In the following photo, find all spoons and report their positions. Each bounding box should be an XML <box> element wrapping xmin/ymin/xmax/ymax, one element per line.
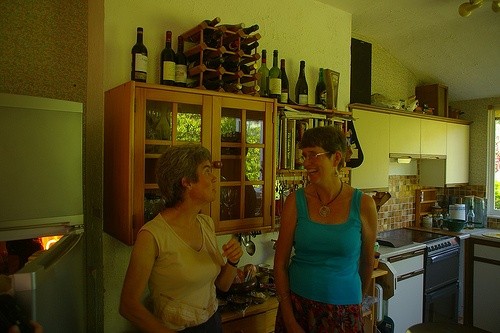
<box><xmin>245</xmin><ymin>235</ymin><xmax>256</xmax><ymax>256</ymax></box>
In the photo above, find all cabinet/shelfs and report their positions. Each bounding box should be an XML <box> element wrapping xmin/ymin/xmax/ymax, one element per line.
<box><xmin>220</xmin><ymin>269</ymin><xmax>388</xmax><ymax>333</ymax></box>
<box><xmin>181</xmin><ymin>23</ymin><xmax>262</xmax><ymax>96</ymax></box>
<box><xmin>461</xmin><ymin>237</ymin><xmax>500</xmax><ymax>333</ymax></box>
<box><xmin>104</xmin><ymin>80</ymin><xmax>278</xmax><ymax>247</ymax></box>
<box><xmin>273</xmin><ymin>103</ymin><xmax>352</xmax><ymax>227</ymax></box>
<box><xmin>348</xmin><ymin>103</ymin><xmax>474</xmax><ymax>190</ymax></box>
<box><xmin>381</xmin><ymin>249</ymin><xmax>425</xmax><ymax>333</ymax></box>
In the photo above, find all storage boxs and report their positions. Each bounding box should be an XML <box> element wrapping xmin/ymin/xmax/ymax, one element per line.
<box><xmin>416</xmin><ymin>84</ymin><xmax>449</xmax><ymax>116</ymax></box>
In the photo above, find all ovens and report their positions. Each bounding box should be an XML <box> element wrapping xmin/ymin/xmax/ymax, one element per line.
<box><xmin>422</xmin><ymin>248</ymin><xmax>459</xmax><ymax>333</ymax></box>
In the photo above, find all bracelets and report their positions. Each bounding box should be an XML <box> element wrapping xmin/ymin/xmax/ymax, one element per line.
<box><xmin>227</xmin><ymin>258</ymin><xmax>240</xmax><ymax>268</ymax></box>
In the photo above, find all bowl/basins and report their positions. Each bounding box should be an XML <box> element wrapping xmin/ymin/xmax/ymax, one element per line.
<box><xmin>446</xmin><ymin>219</ymin><xmax>466</xmax><ymax>231</ymax></box>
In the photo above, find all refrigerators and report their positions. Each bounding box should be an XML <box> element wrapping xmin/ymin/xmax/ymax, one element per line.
<box><xmin>0</xmin><ymin>92</ymin><xmax>87</xmax><ymax>333</ymax></box>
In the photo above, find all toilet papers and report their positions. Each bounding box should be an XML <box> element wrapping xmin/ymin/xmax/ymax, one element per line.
<box><xmin>448</xmin><ymin>203</ymin><xmax>466</xmax><ymax>220</ymax></box>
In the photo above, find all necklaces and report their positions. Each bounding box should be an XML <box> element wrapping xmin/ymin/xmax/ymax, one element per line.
<box><xmin>313</xmin><ymin>179</ymin><xmax>343</xmax><ymax>217</ymax></box>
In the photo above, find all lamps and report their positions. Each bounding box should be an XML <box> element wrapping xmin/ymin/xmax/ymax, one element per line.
<box><xmin>459</xmin><ymin>0</ymin><xmax>500</xmax><ymax>17</ymax></box>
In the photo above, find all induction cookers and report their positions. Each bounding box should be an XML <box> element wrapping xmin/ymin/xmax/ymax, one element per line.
<box><xmin>375</xmin><ymin>227</ymin><xmax>459</xmax><ymax>257</ymax></box>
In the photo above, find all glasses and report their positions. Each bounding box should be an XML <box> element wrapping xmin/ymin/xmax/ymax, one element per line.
<box><xmin>301</xmin><ymin>151</ymin><xmax>334</xmax><ymax>161</ymax></box>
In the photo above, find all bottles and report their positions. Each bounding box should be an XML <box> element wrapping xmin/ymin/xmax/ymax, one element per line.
<box><xmin>314</xmin><ymin>68</ymin><xmax>327</xmax><ymax>107</ymax></box>
<box><xmin>466</xmin><ymin>206</ymin><xmax>476</xmax><ymax>228</ymax></box>
<box><xmin>258</xmin><ymin>50</ymin><xmax>270</xmax><ymax>98</ymax></box>
<box><xmin>187</xmin><ymin>17</ymin><xmax>261</xmax><ymax>96</ymax></box>
<box><xmin>130</xmin><ymin>26</ymin><xmax>148</xmax><ymax>83</ymax></box>
<box><xmin>295</xmin><ymin>60</ymin><xmax>308</xmax><ymax>105</ymax></box>
<box><xmin>280</xmin><ymin>59</ymin><xmax>290</xmax><ymax>103</ymax></box>
<box><xmin>175</xmin><ymin>35</ymin><xmax>187</xmax><ymax>86</ymax></box>
<box><xmin>268</xmin><ymin>49</ymin><xmax>282</xmax><ymax>100</ymax></box>
<box><xmin>159</xmin><ymin>30</ymin><xmax>175</xmax><ymax>85</ymax></box>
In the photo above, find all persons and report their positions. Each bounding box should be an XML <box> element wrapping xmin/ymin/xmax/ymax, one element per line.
<box><xmin>273</xmin><ymin>126</ymin><xmax>378</xmax><ymax>333</ymax></box>
<box><xmin>118</xmin><ymin>144</ymin><xmax>243</xmax><ymax>333</ymax></box>
